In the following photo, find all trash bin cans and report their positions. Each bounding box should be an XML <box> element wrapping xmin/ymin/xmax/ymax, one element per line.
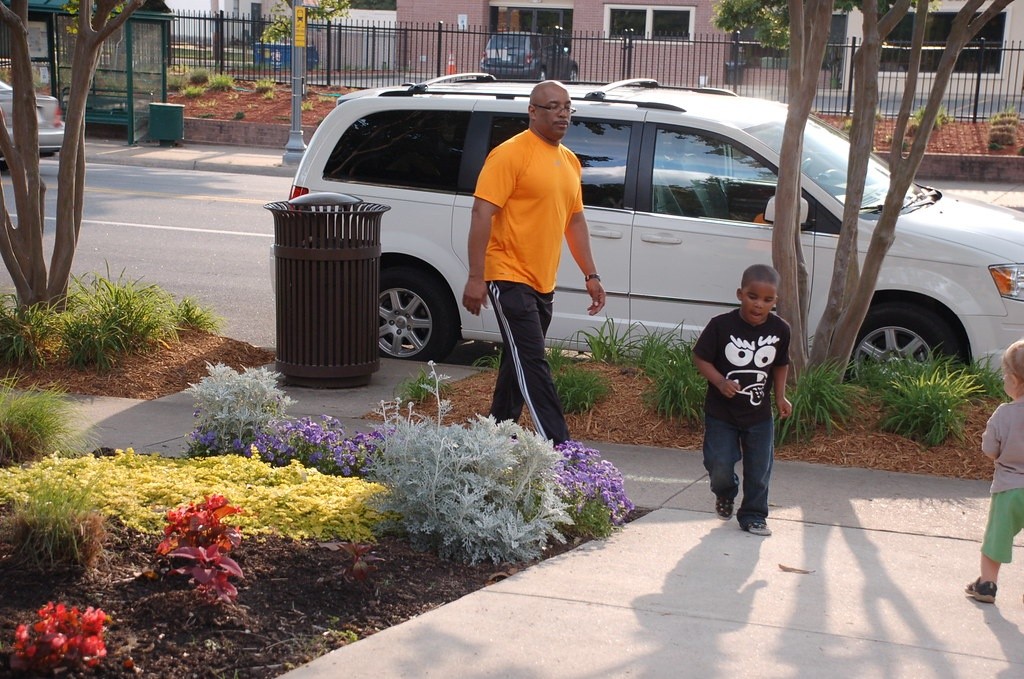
<box><xmin>263</xmin><ymin>190</ymin><xmax>393</xmax><ymax>392</ymax></box>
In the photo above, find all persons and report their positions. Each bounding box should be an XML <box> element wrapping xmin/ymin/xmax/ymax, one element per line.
<box><xmin>461</xmin><ymin>81</ymin><xmax>605</xmax><ymax>446</ymax></box>
<box><xmin>963</xmin><ymin>339</ymin><xmax>1024</xmax><ymax>604</ymax></box>
<box><xmin>691</xmin><ymin>265</ymin><xmax>793</xmax><ymax>535</ymax></box>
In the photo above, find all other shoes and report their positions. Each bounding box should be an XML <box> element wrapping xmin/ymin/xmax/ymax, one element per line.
<box><xmin>747</xmin><ymin>522</ymin><xmax>772</xmax><ymax>535</ymax></box>
<box><xmin>964</xmin><ymin>577</ymin><xmax>998</xmax><ymax>603</ymax></box>
<box><xmin>715</xmin><ymin>473</ymin><xmax>739</xmax><ymax>519</ymax></box>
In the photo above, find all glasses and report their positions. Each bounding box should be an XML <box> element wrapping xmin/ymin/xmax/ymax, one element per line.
<box><xmin>533</xmin><ymin>103</ymin><xmax>576</xmax><ymax>114</ymax></box>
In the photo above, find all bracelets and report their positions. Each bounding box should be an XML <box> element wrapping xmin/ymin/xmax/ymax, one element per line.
<box><xmin>585</xmin><ymin>273</ymin><xmax>602</xmax><ymax>282</ymax></box>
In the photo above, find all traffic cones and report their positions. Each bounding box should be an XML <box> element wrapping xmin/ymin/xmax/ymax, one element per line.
<box><xmin>445</xmin><ymin>52</ymin><xmax>456</xmax><ymax>75</ymax></box>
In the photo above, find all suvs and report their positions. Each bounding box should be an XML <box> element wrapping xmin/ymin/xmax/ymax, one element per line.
<box><xmin>287</xmin><ymin>72</ymin><xmax>1023</xmax><ymax>386</ymax></box>
<box><xmin>482</xmin><ymin>30</ymin><xmax>579</xmax><ymax>83</ymax></box>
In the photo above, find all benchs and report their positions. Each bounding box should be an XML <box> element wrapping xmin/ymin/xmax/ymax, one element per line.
<box><xmin>61</xmin><ymin>85</ymin><xmax>153</xmax><ymax>137</ymax></box>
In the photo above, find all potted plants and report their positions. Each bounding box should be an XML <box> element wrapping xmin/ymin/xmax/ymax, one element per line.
<box><xmin>827</xmin><ymin>48</ymin><xmax>842</xmax><ymax>88</ymax></box>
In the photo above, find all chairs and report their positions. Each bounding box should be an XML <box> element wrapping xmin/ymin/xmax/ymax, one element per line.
<box><xmin>654</xmin><ymin>157</ymin><xmax>730</xmax><ymax>219</ymax></box>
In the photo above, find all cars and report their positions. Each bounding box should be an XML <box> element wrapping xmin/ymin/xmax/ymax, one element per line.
<box><xmin>0</xmin><ymin>80</ymin><xmax>65</xmax><ymax>170</ymax></box>
